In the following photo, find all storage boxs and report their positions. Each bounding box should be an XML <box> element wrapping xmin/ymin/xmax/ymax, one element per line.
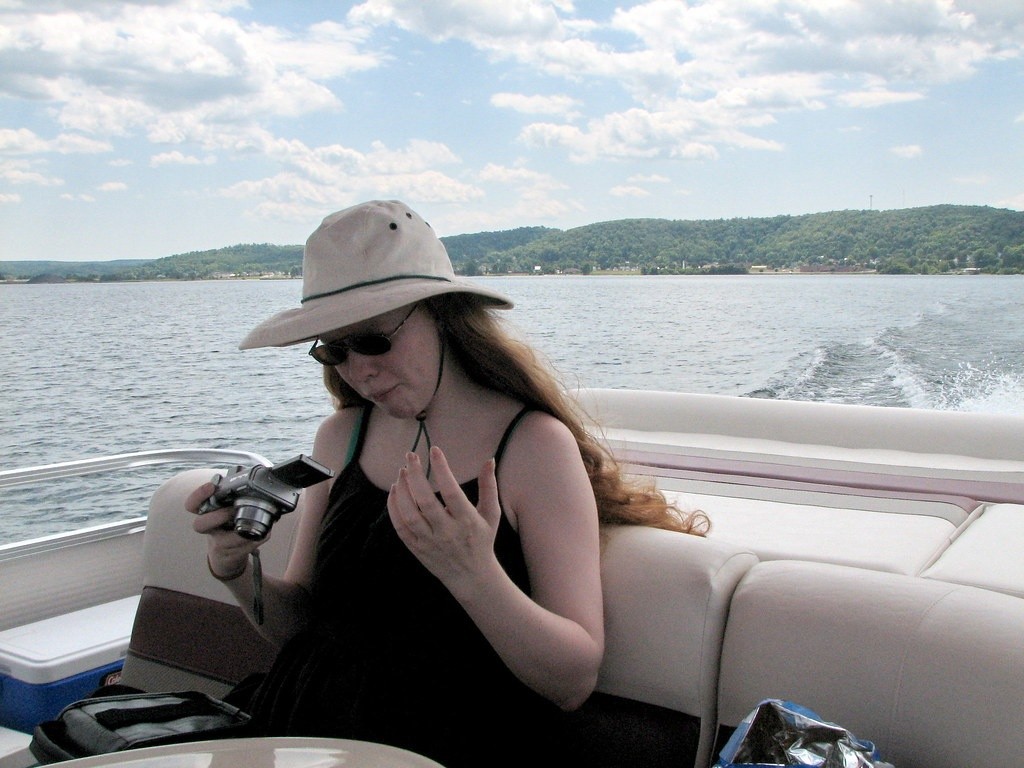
<box><xmin>0</xmin><ymin>593</ymin><xmax>140</xmax><ymax>734</ymax></box>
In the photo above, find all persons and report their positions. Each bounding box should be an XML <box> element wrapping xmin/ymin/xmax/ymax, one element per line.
<box><xmin>185</xmin><ymin>200</ymin><xmax>712</xmax><ymax>768</ymax></box>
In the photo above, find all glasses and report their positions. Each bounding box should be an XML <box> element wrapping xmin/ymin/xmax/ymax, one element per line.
<box><xmin>308</xmin><ymin>302</ymin><xmax>421</xmax><ymax>366</ymax></box>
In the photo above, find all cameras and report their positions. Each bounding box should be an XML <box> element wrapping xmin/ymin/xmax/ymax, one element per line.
<box><xmin>206</xmin><ymin>453</ymin><xmax>334</xmax><ymax>541</ymax></box>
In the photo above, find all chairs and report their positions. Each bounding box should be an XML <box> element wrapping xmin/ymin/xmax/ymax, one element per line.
<box><xmin>120</xmin><ymin>468</ymin><xmax>303</xmax><ymax>697</ymax></box>
<box><xmin>522</xmin><ymin>523</ymin><xmax>758</xmax><ymax>768</ymax></box>
<box><xmin>708</xmin><ymin>560</ymin><xmax>1024</xmax><ymax>768</ymax></box>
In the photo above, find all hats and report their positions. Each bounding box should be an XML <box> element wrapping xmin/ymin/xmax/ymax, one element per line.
<box><xmin>237</xmin><ymin>199</ymin><xmax>515</xmax><ymax>350</ymax></box>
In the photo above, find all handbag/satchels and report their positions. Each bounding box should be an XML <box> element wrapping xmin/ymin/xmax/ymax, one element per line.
<box><xmin>27</xmin><ymin>689</ymin><xmax>256</xmax><ymax>764</ymax></box>
<box><xmin>707</xmin><ymin>699</ymin><xmax>894</xmax><ymax>768</ymax></box>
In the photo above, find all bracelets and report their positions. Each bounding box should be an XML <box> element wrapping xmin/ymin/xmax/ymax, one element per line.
<box><xmin>207</xmin><ymin>554</ymin><xmax>245</xmax><ymax>580</ymax></box>
